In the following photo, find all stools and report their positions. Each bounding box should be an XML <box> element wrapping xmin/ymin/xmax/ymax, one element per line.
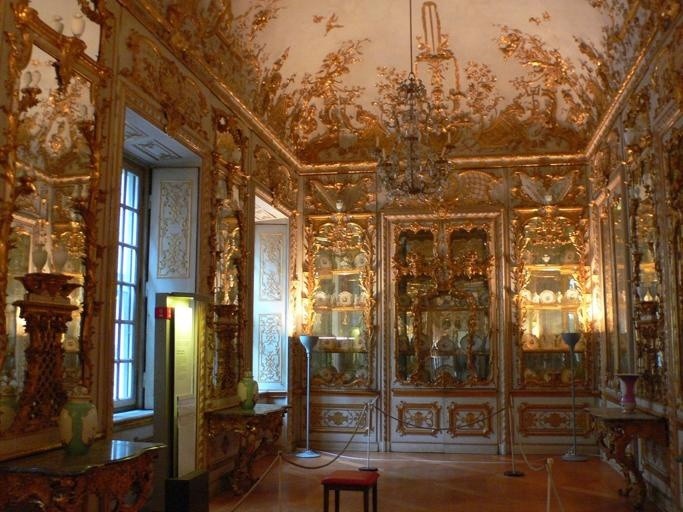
<box><xmin>318</xmin><ymin>468</ymin><xmax>379</xmax><ymax>512</ymax></box>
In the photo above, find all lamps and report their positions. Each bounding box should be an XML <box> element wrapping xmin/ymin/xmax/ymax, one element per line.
<box><xmin>559</xmin><ymin>330</ymin><xmax>589</xmax><ymax>460</ymax></box>
<box><xmin>372</xmin><ymin>1</ymin><xmax>459</xmax><ymax>208</ymax></box>
<box><xmin>292</xmin><ymin>334</ymin><xmax>321</xmax><ymax>459</ymax></box>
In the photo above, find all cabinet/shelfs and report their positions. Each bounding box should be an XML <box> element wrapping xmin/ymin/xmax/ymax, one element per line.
<box><xmin>306</xmin><ymin>268</ymin><xmax>368</xmax><ymax>354</ymax></box>
<box><xmin>518</xmin><ymin>262</ymin><xmax>591</xmax><ymax>354</ymax></box>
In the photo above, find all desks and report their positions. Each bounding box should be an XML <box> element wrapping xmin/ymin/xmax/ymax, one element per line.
<box><xmin>588</xmin><ymin>407</ymin><xmax>668</xmax><ymax>511</ymax></box>
<box><xmin>0</xmin><ymin>436</ymin><xmax>170</xmax><ymax>511</ymax></box>
<box><xmin>203</xmin><ymin>400</ymin><xmax>292</xmax><ymax>498</ymax></box>
